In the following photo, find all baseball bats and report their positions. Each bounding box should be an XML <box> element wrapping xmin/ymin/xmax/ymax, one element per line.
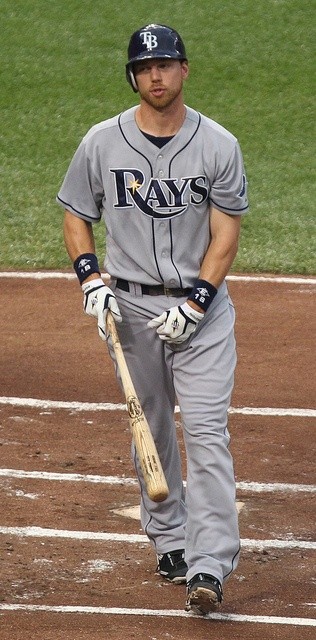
<box><xmin>106</xmin><ymin>311</ymin><xmax>170</xmax><ymax>503</ymax></box>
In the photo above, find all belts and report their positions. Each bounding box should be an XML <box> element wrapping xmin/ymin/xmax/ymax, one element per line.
<box><xmin>115</xmin><ymin>279</ymin><xmax>193</xmax><ymax>298</ymax></box>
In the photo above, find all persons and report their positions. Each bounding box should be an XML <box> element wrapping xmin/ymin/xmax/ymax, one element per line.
<box><xmin>55</xmin><ymin>23</ymin><xmax>251</xmax><ymax>615</ymax></box>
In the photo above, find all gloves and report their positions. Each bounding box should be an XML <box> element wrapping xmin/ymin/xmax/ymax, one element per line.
<box><xmin>83</xmin><ymin>279</ymin><xmax>122</xmax><ymax>342</ymax></box>
<box><xmin>147</xmin><ymin>301</ymin><xmax>204</xmax><ymax>345</ymax></box>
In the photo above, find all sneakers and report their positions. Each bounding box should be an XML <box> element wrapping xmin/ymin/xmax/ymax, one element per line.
<box><xmin>185</xmin><ymin>573</ymin><xmax>223</xmax><ymax>616</ymax></box>
<box><xmin>156</xmin><ymin>549</ymin><xmax>188</xmax><ymax>584</ymax></box>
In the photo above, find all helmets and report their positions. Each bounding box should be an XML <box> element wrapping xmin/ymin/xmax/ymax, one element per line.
<box><xmin>125</xmin><ymin>24</ymin><xmax>185</xmax><ymax>93</ymax></box>
